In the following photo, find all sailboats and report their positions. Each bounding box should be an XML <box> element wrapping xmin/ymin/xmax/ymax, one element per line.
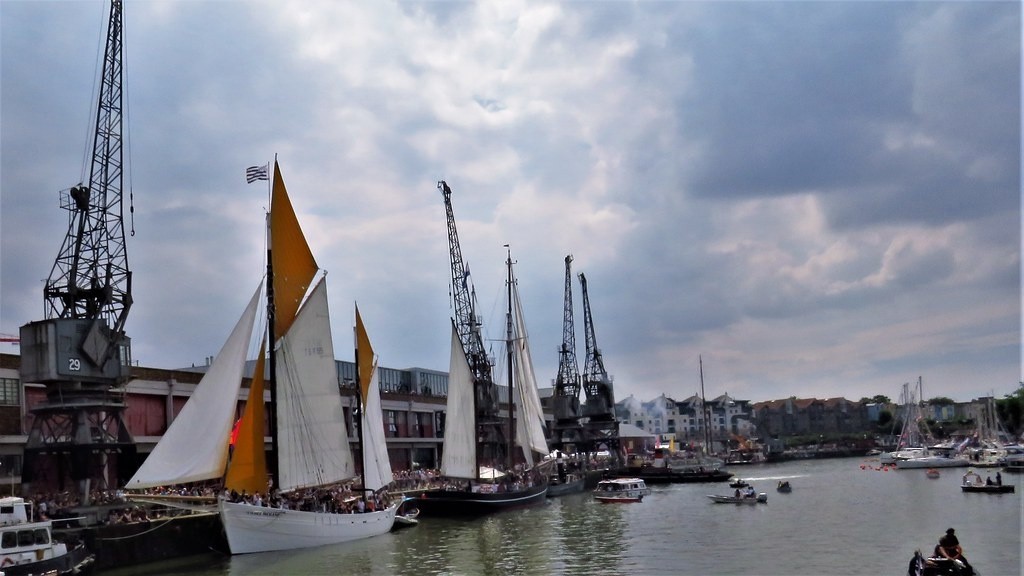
<box><xmin>116</xmin><ymin>152</ymin><xmax>735</xmax><ymax>554</ymax></box>
<box><xmin>880</xmin><ymin>376</ymin><xmax>1024</xmax><ymax>473</ymax></box>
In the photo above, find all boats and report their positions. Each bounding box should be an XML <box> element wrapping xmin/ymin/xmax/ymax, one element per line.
<box><xmin>706</xmin><ymin>492</ymin><xmax>767</xmax><ymax>504</ymax></box>
<box><xmin>729</xmin><ymin>483</ymin><xmax>749</xmax><ymax>488</ymax></box>
<box><xmin>592</xmin><ymin>478</ymin><xmax>651</xmax><ymax>502</ymax></box>
<box><xmin>0</xmin><ymin>494</ymin><xmax>221</xmax><ymax>575</ymax></box>
<box><xmin>865</xmin><ymin>449</ymin><xmax>881</xmax><ymax>456</ymax></box>
<box><xmin>777</xmin><ymin>487</ymin><xmax>792</xmax><ymax>493</ymax></box>
<box><xmin>960</xmin><ymin>470</ymin><xmax>1015</xmax><ymax>494</ymax></box>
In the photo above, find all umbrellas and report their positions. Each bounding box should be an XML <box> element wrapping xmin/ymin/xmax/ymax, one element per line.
<box><xmin>544</xmin><ymin>449</ymin><xmax>569</xmax><ymax>471</ymax></box>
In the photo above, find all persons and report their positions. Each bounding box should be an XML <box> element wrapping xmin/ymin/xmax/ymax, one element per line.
<box><xmin>986</xmin><ymin>477</ymin><xmax>993</xmax><ymax>485</ymax></box>
<box><xmin>933</xmin><ymin>528</ymin><xmax>962</xmax><ymax>561</ymax></box>
<box><xmin>1</xmin><ymin>485</ymin><xmax>217</xmax><ymax>526</ymax></box>
<box><xmin>352</xmin><ymin>469</ymin><xmax>441</xmax><ymax>491</ymax></box>
<box><xmin>444</xmin><ymin>461</ymin><xmax>531</xmax><ymax>495</ymax></box>
<box><xmin>743</xmin><ymin>486</ymin><xmax>756</xmax><ymax>499</ymax></box>
<box><xmin>224</xmin><ymin>486</ymin><xmax>395</xmax><ymax>514</ymax></box>
<box><xmin>995</xmin><ymin>472</ymin><xmax>1002</xmax><ymax>485</ymax></box>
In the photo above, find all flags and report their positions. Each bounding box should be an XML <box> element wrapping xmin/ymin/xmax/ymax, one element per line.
<box><xmin>246</xmin><ymin>165</ymin><xmax>269</xmax><ymax>184</ymax></box>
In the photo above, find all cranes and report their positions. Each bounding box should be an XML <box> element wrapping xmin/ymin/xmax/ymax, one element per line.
<box><xmin>19</xmin><ymin>0</ymin><xmax>139</xmax><ymax>504</ymax></box>
<box><xmin>550</xmin><ymin>248</ymin><xmax>623</xmax><ymax>461</ymax></box>
<box><xmin>436</xmin><ymin>178</ymin><xmax>503</xmax><ymax>426</ymax></box>
